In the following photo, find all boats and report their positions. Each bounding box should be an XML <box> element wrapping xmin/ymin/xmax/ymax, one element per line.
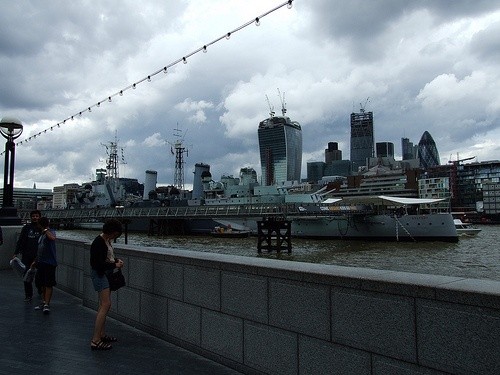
<box><xmin>209</xmin><ymin>229</ymin><xmax>251</xmax><ymax>238</ymax></box>
<box><xmin>456</xmin><ymin>228</ymin><xmax>482</xmax><ymax>236</ymax></box>
<box><xmin>453</xmin><ymin>218</ymin><xmax>472</xmax><ymax>229</ymax></box>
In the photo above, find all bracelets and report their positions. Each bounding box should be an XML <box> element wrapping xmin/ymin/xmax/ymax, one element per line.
<box><xmin>44</xmin><ymin>228</ymin><xmax>47</xmax><ymax>232</ymax></box>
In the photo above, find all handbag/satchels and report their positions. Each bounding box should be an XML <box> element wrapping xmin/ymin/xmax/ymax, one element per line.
<box><xmin>10</xmin><ymin>257</ymin><xmax>26</xmax><ymax>272</ymax></box>
<box><xmin>24</xmin><ymin>268</ymin><xmax>35</xmax><ymax>282</ymax></box>
<box><xmin>107</xmin><ymin>271</ymin><xmax>126</xmax><ymax>291</ymax></box>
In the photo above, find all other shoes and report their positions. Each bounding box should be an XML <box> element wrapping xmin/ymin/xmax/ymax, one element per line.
<box><xmin>35</xmin><ymin>302</ymin><xmax>46</xmax><ymax>310</ymax></box>
<box><xmin>23</xmin><ymin>295</ymin><xmax>33</xmax><ymax>303</ymax></box>
<box><xmin>43</xmin><ymin>304</ymin><xmax>50</xmax><ymax>312</ymax></box>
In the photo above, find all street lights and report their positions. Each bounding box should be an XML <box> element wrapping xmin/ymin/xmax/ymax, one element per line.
<box><xmin>0</xmin><ymin>115</ymin><xmax>24</xmax><ymax>227</ymax></box>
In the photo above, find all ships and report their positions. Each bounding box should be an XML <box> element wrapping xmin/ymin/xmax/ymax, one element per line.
<box><xmin>34</xmin><ymin>128</ymin><xmax>460</xmax><ymax>244</ymax></box>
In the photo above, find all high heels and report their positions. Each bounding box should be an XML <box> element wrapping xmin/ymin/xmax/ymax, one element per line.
<box><xmin>90</xmin><ymin>340</ymin><xmax>112</xmax><ymax>352</ymax></box>
<box><xmin>100</xmin><ymin>335</ymin><xmax>117</xmax><ymax>342</ymax></box>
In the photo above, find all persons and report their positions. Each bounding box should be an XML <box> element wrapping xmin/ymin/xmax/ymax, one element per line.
<box><xmin>12</xmin><ymin>211</ymin><xmax>43</xmax><ymax>302</ymax></box>
<box><xmin>31</xmin><ymin>217</ymin><xmax>56</xmax><ymax>313</ymax></box>
<box><xmin>90</xmin><ymin>218</ymin><xmax>123</xmax><ymax>351</ymax></box>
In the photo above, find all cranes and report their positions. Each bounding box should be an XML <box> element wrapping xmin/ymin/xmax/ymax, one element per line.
<box><xmin>359</xmin><ymin>96</ymin><xmax>370</xmax><ymax>113</ymax></box>
<box><xmin>263</xmin><ymin>88</ymin><xmax>288</xmax><ymax>118</ymax></box>
